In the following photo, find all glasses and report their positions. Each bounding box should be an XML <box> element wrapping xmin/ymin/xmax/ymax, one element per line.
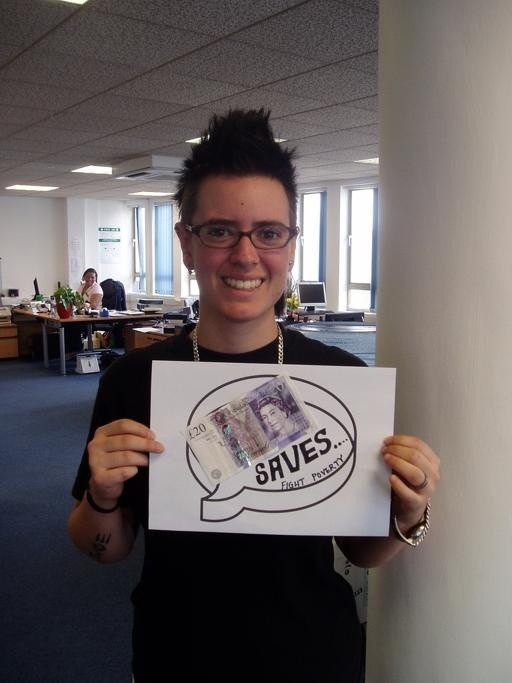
<box><xmin>185</xmin><ymin>221</ymin><xmax>297</xmax><ymax>250</ymax></box>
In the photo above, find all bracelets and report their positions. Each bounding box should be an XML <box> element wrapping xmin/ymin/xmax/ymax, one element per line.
<box><xmin>83</xmin><ymin>487</ymin><xmax>122</xmax><ymax>516</ymax></box>
<box><xmin>394</xmin><ymin>501</ymin><xmax>432</xmax><ymax>550</ymax></box>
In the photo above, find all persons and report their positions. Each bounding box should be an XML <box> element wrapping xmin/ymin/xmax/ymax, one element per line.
<box><xmin>67</xmin><ymin>266</ymin><xmax>105</xmax><ymax>361</ymax></box>
<box><xmin>255</xmin><ymin>396</ymin><xmax>298</xmax><ymax>437</ymax></box>
<box><xmin>66</xmin><ymin>101</ymin><xmax>442</xmax><ymax>681</ymax></box>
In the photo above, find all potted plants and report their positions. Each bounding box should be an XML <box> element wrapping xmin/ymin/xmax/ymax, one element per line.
<box><xmin>53</xmin><ymin>285</ymin><xmax>85</xmax><ymax>319</ymax></box>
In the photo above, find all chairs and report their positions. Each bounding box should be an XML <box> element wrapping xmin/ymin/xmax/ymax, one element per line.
<box><xmin>88</xmin><ymin>278</ymin><xmax>127</xmax><ymax>350</ymax></box>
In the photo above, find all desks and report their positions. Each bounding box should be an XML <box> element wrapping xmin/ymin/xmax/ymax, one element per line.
<box><xmin>12</xmin><ymin>306</ymin><xmax>178</xmax><ymax>376</ymax></box>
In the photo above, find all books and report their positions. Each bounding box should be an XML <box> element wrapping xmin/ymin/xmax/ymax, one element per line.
<box><xmin>132</xmin><ymin>326</ymin><xmax>160</xmax><ymax>334</ymax></box>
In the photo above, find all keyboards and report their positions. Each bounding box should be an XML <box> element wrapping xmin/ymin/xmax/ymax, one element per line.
<box><xmin>298</xmin><ymin>310</ymin><xmax>334</xmax><ymax>315</ymax></box>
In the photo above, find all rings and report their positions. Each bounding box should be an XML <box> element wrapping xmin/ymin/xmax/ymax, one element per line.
<box><xmin>414</xmin><ymin>472</ymin><xmax>430</xmax><ymax>489</ymax></box>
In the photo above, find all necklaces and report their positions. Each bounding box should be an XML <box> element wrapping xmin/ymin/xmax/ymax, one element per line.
<box><xmin>190</xmin><ymin>319</ymin><xmax>288</xmax><ymax>368</ymax></box>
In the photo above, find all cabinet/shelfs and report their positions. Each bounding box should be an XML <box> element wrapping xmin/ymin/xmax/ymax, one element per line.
<box><xmin>0</xmin><ymin>323</ymin><xmax>20</xmax><ymax>361</ymax></box>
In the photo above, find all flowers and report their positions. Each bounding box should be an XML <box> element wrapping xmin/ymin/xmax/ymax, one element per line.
<box><xmin>285</xmin><ymin>293</ymin><xmax>301</xmax><ymax>319</ymax></box>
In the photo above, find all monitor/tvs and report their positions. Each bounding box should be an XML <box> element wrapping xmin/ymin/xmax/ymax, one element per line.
<box><xmin>325</xmin><ymin>312</ymin><xmax>364</xmax><ymax>322</ymax></box>
<box><xmin>297</xmin><ymin>281</ymin><xmax>327</xmax><ymax>312</ymax></box>
<box><xmin>32</xmin><ymin>278</ymin><xmax>40</xmax><ymax>300</ymax></box>
